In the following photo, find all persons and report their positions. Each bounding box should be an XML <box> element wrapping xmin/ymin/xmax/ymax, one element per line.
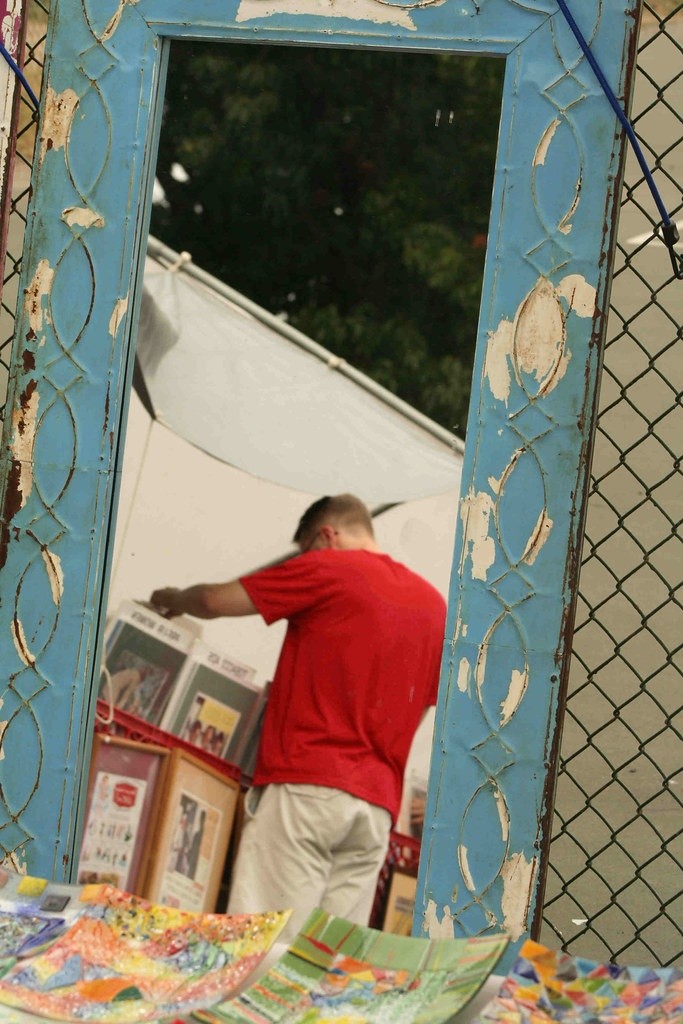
<box><xmin>152</xmin><ymin>493</ymin><xmax>449</xmax><ymax>930</ymax></box>
<box><xmin>101</xmin><ymin>666</ymin><xmax>146</xmax><ymax>709</ymax></box>
<box><xmin>171</xmin><ymin>810</ymin><xmax>207</xmax><ymax>879</ymax></box>
<box><xmin>186</xmin><ymin>722</ymin><xmax>226</xmax><ymax>754</ymax></box>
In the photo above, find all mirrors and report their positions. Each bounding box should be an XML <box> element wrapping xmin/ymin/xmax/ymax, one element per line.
<box><xmin>0</xmin><ymin>0</ymin><xmax>639</xmax><ymax>985</ymax></box>
